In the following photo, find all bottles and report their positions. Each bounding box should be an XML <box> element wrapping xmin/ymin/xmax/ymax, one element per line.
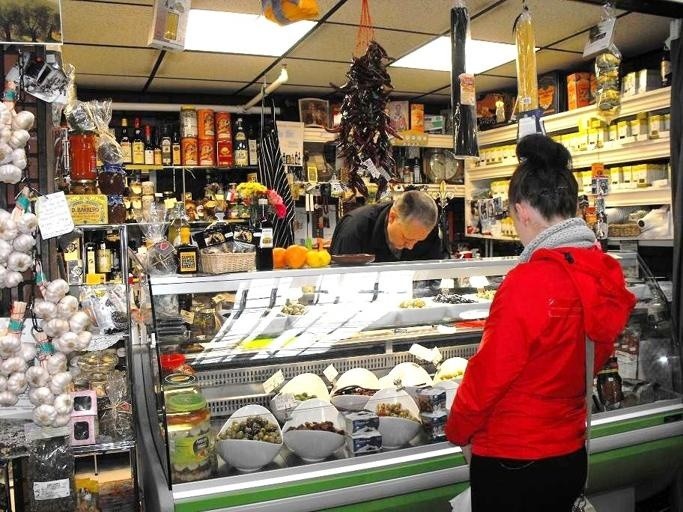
<box><xmin>226</xmin><ymin>183</ymin><xmax>239</xmax><ymax>202</ymax></box>
<box><xmin>160</xmin><ymin>123</ymin><xmax>172</xmax><ymax>164</ymax></box>
<box><xmin>613</xmin><ymin>328</ymin><xmax>639</xmax><ymax>377</ymax></box>
<box><xmin>120</xmin><ymin>118</ymin><xmax>132</xmax><ymax>164</ymax></box>
<box><xmin>132</xmin><ymin>118</ymin><xmax>145</xmax><ymax>164</ymax></box>
<box><xmin>215</xmin><ymin>111</ymin><xmax>231</xmax><ymax>140</ymax></box>
<box><xmin>182</xmin><ymin>136</ymin><xmax>196</xmax><ymax>166</ymax></box>
<box><xmin>90</xmin><ymin>229</ymin><xmax>97</xmax><ymax>244</ymax></box>
<box><xmin>122</xmin><ymin>197</ymin><xmax>129</xmax><ymax>222</ymax></box>
<box><xmin>108</xmin><ymin>195</ymin><xmax>126</xmax><ymax>223</ymax></box>
<box><xmin>254</xmin><ymin>199</ymin><xmax>273</xmax><ymax>270</ymax></box>
<box><xmin>180</xmin><ymin>105</ymin><xmax>197</xmax><ymax>137</ymax></box>
<box><xmin>175</xmin><ymin>227</ymin><xmax>198</xmax><ymax>272</ymax></box>
<box><xmin>413</xmin><ymin>157</ymin><xmax>420</xmax><ymax>183</ymax></box>
<box><xmin>144</xmin><ymin>124</ymin><xmax>154</xmax><ymax>164</ymax></box>
<box><xmin>312</xmin><ymin>193</ymin><xmax>325</xmax><ymax>238</ymax></box>
<box><xmin>142</xmin><ymin>182</ymin><xmax>154</xmax><ymax>195</ymax></box>
<box><xmin>197</xmin><ymin>139</ymin><xmax>215</xmax><ymax>167</ymax></box>
<box><xmin>239</xmin><ymin>202</ymin><xmax>250</xmax><ymax>218</ymax></box>
<box><xmin>129</xmin><ymin>196</ymin><xmax>142</xmax><ymax>223</ymax></box>
<box><xmin>234</xmin><ymin>118</ymin><xmax>249</xmax><ymax>166</ymax></box>
<box><xmin>98</xmin><ymin>164</ymin><xmax>126</xmax><ymax>195</ymax></box>
<box><xmin>96</xmin><ymin>241</ymin><xmax>110</xmax><ymax>281</ymax></box>
<box><xmin>164</xmin><ymin>192</ymin><xmax>173</xmax><ymax>200</ymax></box>
<box><xmin>248</xmin><ymin>127</ymin><xmax>258</xmax><ymax>165</ymax></box>
<box><xmin>87</xmin><ymin>245</ymin><xmax>95</xmax><ymax>274</ymax></box>
<box><xmin>403</xmin><ymin>165</ymin><xmax>411</xmax><ymax>182</ymax></box>
<box><xmin>227</xmin><ymin>203</ymin><xmax>238</xmax><ymax>219</ymax></box>
<box><xmin>661</xmin><ymin>40</ymin><xmax>672</xmax><ymax>85</ymax></box>
<box><xmin>152</xmin><ymin>127</ymin><xmax>162</xmax><ymax>164</ymax></box>
<box><xmin>172</xmin><ymin>125</ymin><xmax>181</xmax><ymax>165</ymax></box>
<box><xmin>130</xmin><ymin>182</ymin><xmax>141</xmax><ymax>196</ymax></box>
<box><xmin>198</xmin><ymin>106</ymin><xmax>213</xmax><ymax>139</ymax></box>
<box><xmin>217</xmin><ymin>140</ymin><xmax>232</xmax><ymax>166</ymax></box>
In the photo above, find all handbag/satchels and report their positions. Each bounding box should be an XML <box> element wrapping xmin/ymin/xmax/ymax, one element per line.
<box><xmin>573</xmin><ymin>334</ymin><xmax>596</xmax><ymax>512</ymax></box>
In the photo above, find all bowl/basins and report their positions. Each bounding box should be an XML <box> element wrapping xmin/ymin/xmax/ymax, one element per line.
<box><xmin>363</xmin><ymin>386</ymin><xmax>423</xmax><ymax>449</ymax></box>
<box><xmin>388</xmin><ymin>362</ymin><xmax>433</xmax><ymax>388</ymax></box>
<box><xmin>433</xmin><ymin>357</ymin><xmax>468</xmax><ymax>385</ymax></box>
<box><xmin>282</xmin><ymin>397</ymin><xmax>348</xmax><ymax>462</ymax></box>
<box><xmin>329</xmin><ymin>368</ymin><xmax>380</xmax><ymax>411</ymax></box>
<box><xmin>214</xmin><ymin>404</ymin><xmax>284</xmax><ymax>472</ymax></box>
<box><xmin>270</xmin><ymin>373</ymin><xmax>330</xmax><ymax>423</ymax></box>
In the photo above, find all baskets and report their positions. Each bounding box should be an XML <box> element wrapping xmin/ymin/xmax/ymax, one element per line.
<box><xmin>198</xmin><ymin>250</ymin><xmax>257</xmax><ymax>274</ymax></box>
<box><xmin>608</xmin><ymin>223</ymin><xmax>640</xmax><ymax>237</ymax></box>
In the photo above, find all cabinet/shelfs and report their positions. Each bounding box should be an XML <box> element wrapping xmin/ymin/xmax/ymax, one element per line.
<box><xmin>461</xmin><ymin>84</ymin><xmax>675</xmax><ymax>249</ymax></box>
<box><xmin>274</xmin><ymin>116</ymin><xmax>486</xmax><ymax>263</ymax></box>
<box><xmin>125</xmin><ymin>255</ymin><xmax>681</xmax><ymax>512</ymax></box>
<box><xmin>51</xmin><ymin>100</ymin><xmax>280</xmax><ymax>271</ymax></box>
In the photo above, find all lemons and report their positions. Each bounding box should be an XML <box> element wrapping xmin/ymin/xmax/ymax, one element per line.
<box><xmin>306</xmin><ymin>249</ymin><xmax>330</xmax><ymax>267</ymax></box>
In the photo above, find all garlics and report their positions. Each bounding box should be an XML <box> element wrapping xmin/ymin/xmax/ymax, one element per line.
<box><xmin>36</xmin><ymin>278</ymin><xmax>92</xmax><ymax>356</ymax></box>
<box><xmin>24</xmin><ymin>351</ymin><xmax>75</xmax><ymax>428</ymax></box>
<box><xmin>0</xmin><ymin>208</ymin><xmax>37</xmax><ymax>289</ymax></box>
<box><xmin>0</xmin><ymin>334</ymin><xmax>37</xmax><ymax>406</ymax></box>
<box><xmin>0</xmin><ymin>100</ymin><xmax>35</xmax><ymax>185</ymax></box>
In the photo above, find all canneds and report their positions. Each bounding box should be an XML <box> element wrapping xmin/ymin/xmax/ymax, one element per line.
<box><xmin>162</xmin><ymin>373</ymin><xmax>200</xmax><ymax>403</ymax></box>
<box><xmin>163</xmin><ymin>395</ymin><xmax>213</xmax><ymax>481</ymax></box>
<box><xmin>605</xmin><ymin>357</ymin><xmax>618</xmax><ymax>371</ymax></box>
<box><xmin>596</xmin><ymin>369</ymin><xmax>623</xmax><ymax>404</ymax></box>
<box><xmin>201</xmin><ymin>310</ymin><xmax>215</xmax><ymax>333</ymax></box>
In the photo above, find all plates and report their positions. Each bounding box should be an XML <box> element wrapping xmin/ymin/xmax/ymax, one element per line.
<box><xmin>331</xmin><ymin>253</ymin><xmax>375</xmax><ymax>267</ymax></box>
<box><xmin>459</xmin><ymin>308</ymin><xmax>490</xmax><ymax>320</ymax></box>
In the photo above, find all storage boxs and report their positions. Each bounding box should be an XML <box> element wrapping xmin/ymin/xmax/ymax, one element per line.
<box><xmin>605</xmin><ymin>222</ymin><xmax>643</xmax><ymax>238</ymax></box>
<box><xmin>567</xmin><ymin>73</ymin><xmax>590</xmax><ymax>111</ymax></box>
<box><xmin>536</xmin><ymin>68</ymin><xmax>564</xmax><ymax>118</ymax></box>
<box><xmin>196</xmin><ymin>245</ymin><xmax>256</xmax><ymax>276</ymax></box>
<box><xmin>423</xmin><ymin>115</ymin><xmax>444</xmax><ymax>135</ymax></box>
<box><xmin>385</xmin><ymin>101</ymin><xmax>408</xmax><ymax>133</ymax></box>
<box><xmin>410</xmin><ymin>103</ymin><xmax>423</xmax><ymax>134</ymax></box>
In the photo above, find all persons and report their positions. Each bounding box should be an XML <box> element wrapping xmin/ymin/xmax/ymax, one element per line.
<box><xmin>330</xmin><ymin>190</ymin><xmax>444</xmax><ymax>262</ymax></box>
<box><xmin>444</xmin><ymin>133</ymin><xmax>635</xmax><ymax>512</ymax></box>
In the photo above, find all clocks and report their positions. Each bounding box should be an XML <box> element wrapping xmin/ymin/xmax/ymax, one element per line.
<box><xmin>423</xmin><ymin>148</ymin><xmax>465</xmax><ymax>184</ymax></box>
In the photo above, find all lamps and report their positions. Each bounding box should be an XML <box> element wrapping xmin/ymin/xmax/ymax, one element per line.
<box><xmin>182</xmin><ymin>7</ymin><xmax>317</xmax><ymax>58</ymax></box>
<box><xmin>385</xmin><ymin>35</ymin><xmax>541</xmax><ymax>77</ymax></box>
